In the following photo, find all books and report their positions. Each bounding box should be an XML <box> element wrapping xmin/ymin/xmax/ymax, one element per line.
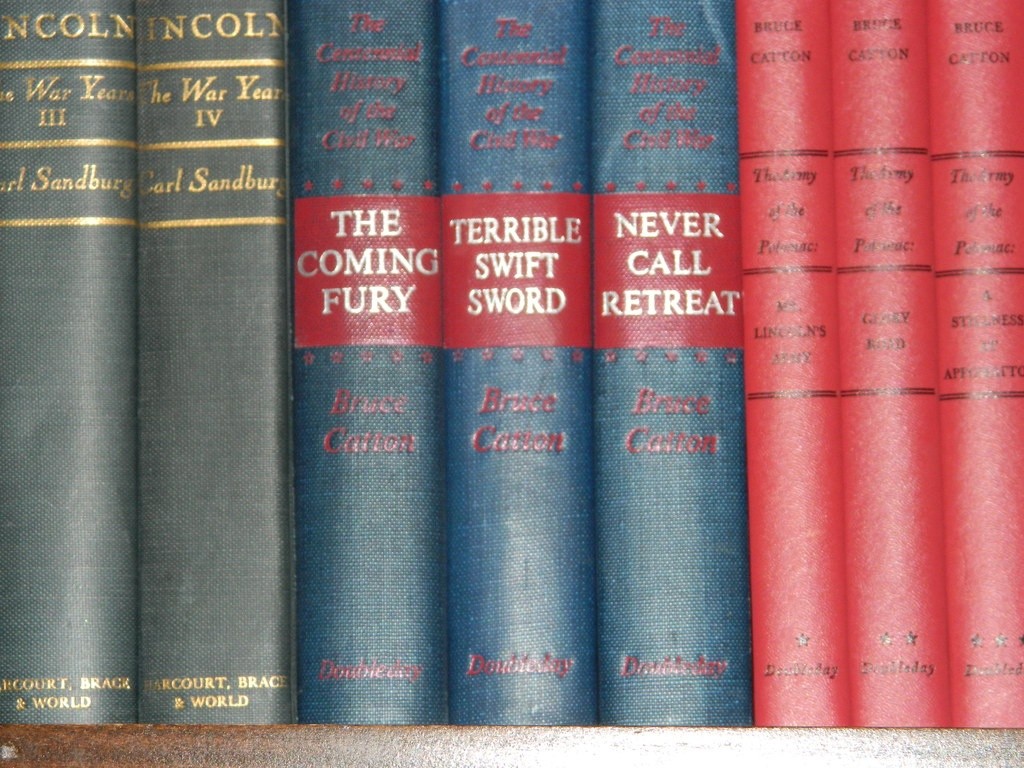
<box><xmin>1</xmin><ymin>1</ymin><xmax>1024</xmax><ymax>728</ymax></box>
